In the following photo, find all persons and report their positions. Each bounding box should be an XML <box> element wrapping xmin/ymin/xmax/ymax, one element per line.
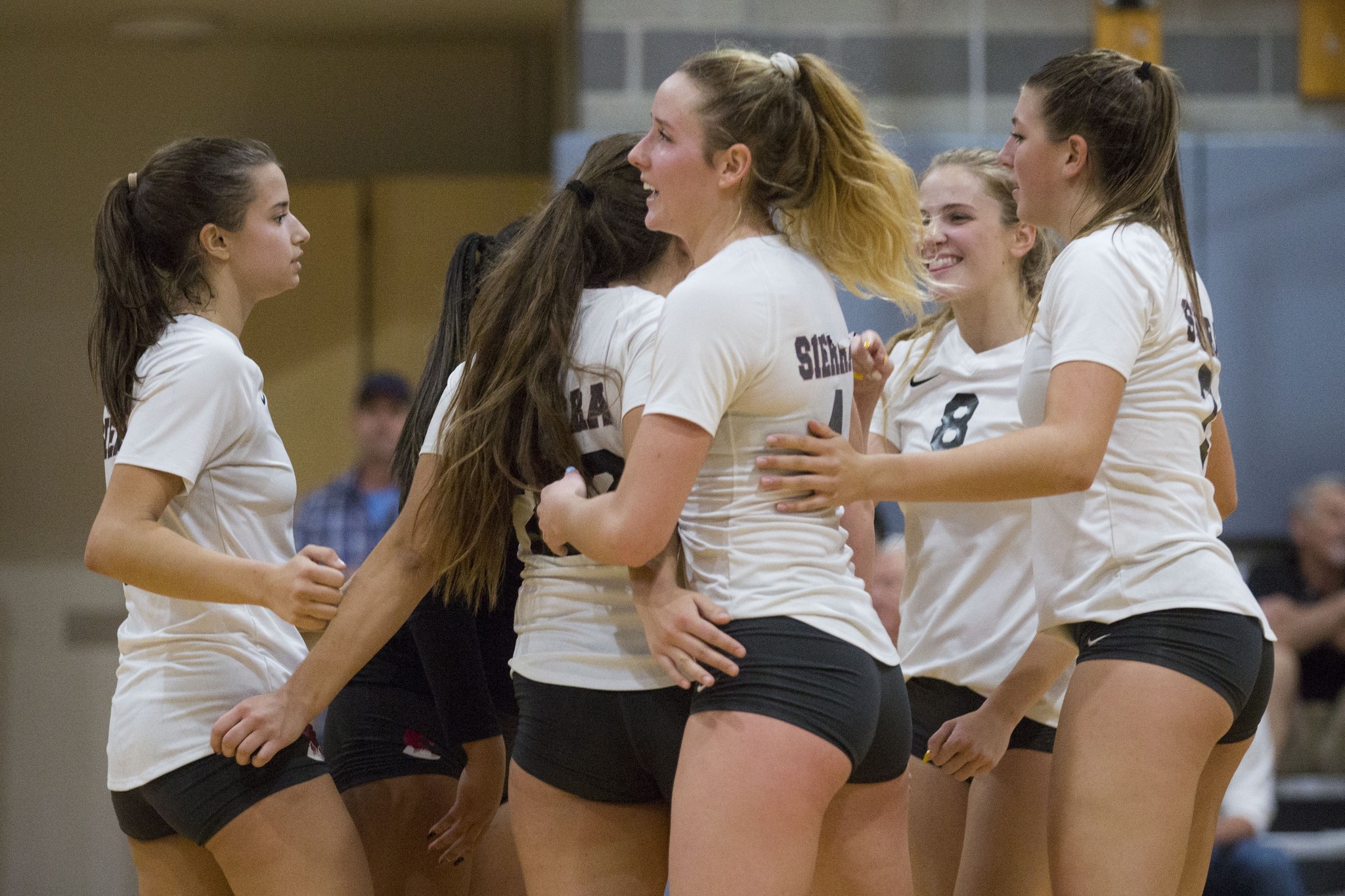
<box><xmin>90</xmin><ymin>137</ymin><xmax>378</xmax><ymax>895</ymax></box>
<box><xmin>208</xmin><ymin>51</ymin><xmax>1345</xmax><ymax>895</ymax></box>
